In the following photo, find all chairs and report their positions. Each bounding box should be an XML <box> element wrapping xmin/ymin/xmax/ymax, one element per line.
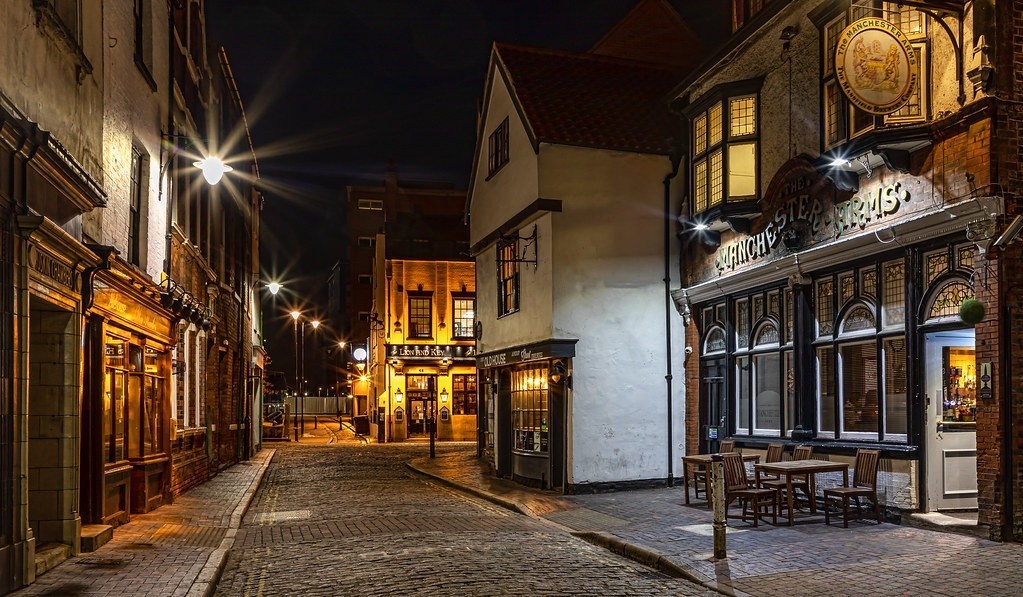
<box><xmin>694</xmin><ymin>440</ymin><xmax>814</xmax><ymax>526</ymax></box>
<box><xmin>824</xmin><ymin>449</ymin><xmax>882</xmax><ymax>526</ymax></box>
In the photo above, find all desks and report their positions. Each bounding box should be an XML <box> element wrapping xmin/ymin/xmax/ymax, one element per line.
<box><xmin>754</xmin><ymin>460</ymin><xmax>849</xmax><ymax>526</ymax></box>
<box><xmin>682</xmin><ymin>454</ymin><xmax>761</xmax><ymax>509</ymax></box>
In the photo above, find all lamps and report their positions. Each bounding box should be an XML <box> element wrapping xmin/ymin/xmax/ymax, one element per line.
<box><xmin>395</xmin><ymin>388</ymin><xmax>403</xmax><ymax>403</ymax></box>
<box><xmin>481</xmin><ymin>374</ymin><xmax>498</xmax><ymax>394</ymax></box>
<box><xmin>441</xmin><ymin>388</ymin><xmax>448</xmax><ymax>403</ymax></box>
<box><xmin>550</xmin><ymin>361</ymin><xmax>573</xmax><ymax>389</ymax></box>
<box><xmin>158</xmin><ymin>278</ymin><xmax>212</xmax><ymax>331</ymax></box>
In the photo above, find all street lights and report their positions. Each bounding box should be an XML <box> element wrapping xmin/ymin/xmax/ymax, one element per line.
<box><xmin>299</xmin><ymin>319</ymin><xmax>323</xmax><ymax>438</ymax></box>
<box><xmin>289</xmin><ymin>310</ymin><xmax>301</xmax><ymax>442</ymax></box>
<box><xmin>334</xmin><ymin>341</ymin><xmax>345</xmax><ymax>418</ymax></box>
